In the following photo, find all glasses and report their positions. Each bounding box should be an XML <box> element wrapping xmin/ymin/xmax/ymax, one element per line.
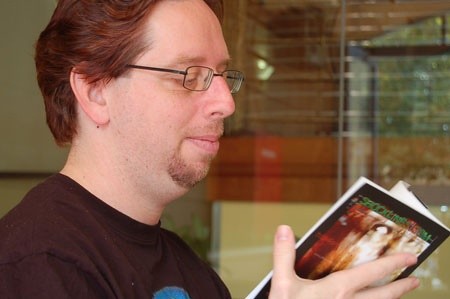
<box><xmin>124</xmin><ymin>64</ymin><xmax>245</xmax><ymax>93</ymax></box>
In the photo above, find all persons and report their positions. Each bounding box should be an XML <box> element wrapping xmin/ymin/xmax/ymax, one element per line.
<box><xmin>0</xmin><ymin>0</ymin><xmax>420</xmax><ymax>299</ymax></box>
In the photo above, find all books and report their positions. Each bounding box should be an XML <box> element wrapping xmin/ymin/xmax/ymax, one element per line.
<box><xmin>245</xmin><ymin>176</ymin><xmax>450</xmax><ymax>299</ymax></box>
